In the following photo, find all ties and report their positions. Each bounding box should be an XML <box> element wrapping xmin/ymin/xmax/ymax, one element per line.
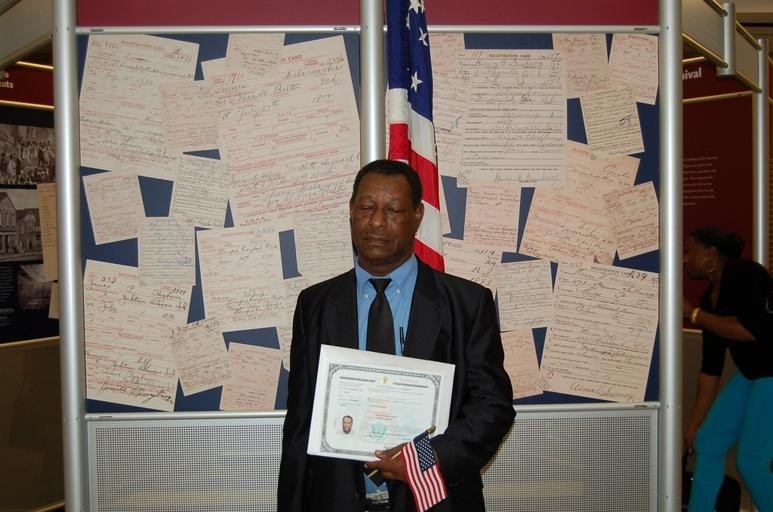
<box><xmin>365</xmin><ymin>278</ymin><xmax>398</xmax><ymax>486</ymax></box>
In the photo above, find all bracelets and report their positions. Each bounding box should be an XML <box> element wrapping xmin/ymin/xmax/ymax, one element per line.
<box><xmin>690</xmin><ymin>306</ymin><xmax>702</xmax><ymax>327</ymax></box>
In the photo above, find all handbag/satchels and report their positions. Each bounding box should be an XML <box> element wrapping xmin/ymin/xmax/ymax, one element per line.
<box><xmin>681</xmin><ymin>445</ymin><xmax>741</xmax><ymax>512</ymax></box>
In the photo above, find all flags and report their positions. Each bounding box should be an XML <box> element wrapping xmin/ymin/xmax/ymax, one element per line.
<box><xmin>400</xmin><ymin>431</ymin><xmax>447</xmax><ymax>511</ymax></box>
<box><xmin>386</xmin><ymin>0</ymin><xmax>445</xmax><ymax>275</ymax></box>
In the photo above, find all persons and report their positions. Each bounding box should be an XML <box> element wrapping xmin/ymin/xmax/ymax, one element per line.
<box><xmin>335</xmin><ymin>414</ymin><xmax>354</xmax><ymax>435</ymax></box>
<box><xmin>0</xmin><ymin>137</ymin><xmax>53</xmax><ymax>185</ymax></box>
<box><xmin>682</xmin><ymin>227</ymin><xmax>772</xmax><ymax>511</ymax></box>
<box><xmin>275</xmin><ymin>159</ymin><xmax>516</xmax><ymax>510</ymax></box>
